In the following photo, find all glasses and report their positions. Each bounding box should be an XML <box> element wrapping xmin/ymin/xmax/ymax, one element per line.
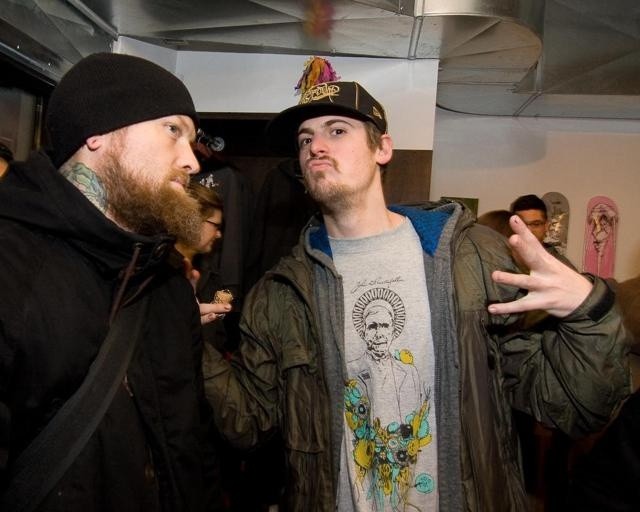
<box><xmin>203</xmin><ymin>218</ymin><xmax>223</xmax><ymax>232</ymax></box>
<box><xmin>524</xmin><ymin>221</ymin><xmax>546</xmax><ymax>226</ymax></box>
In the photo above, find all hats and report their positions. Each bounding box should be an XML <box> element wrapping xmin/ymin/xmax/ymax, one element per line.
<box><xmin>42</xmin><ymin>51</ymin><xmax>198</xmax><ymax>168</ymax></box>
<box><xmin>260</xmin><ymin>81</ymin><xmax>390</xmax><ymax>159</ymax></box>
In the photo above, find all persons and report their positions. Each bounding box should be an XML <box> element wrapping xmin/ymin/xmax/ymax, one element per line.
<box><xmin>174</xmin><ymin>178</ymin><xmax>233</xmax><ymax>362</ymax></box>
<box><xmin>183</xmin><ymin>79</ymin><xmax>638</xmax><ymax>511</ymax></box>
<box><xmin>0</xmin><ymin>143</ymin><xmax>16</xmax><ymax>179</ymax></box>
<box><xmin>474</xmin><ymin>194</ymin><xmax>640</xmax><ymax>512</ymax></box>
<box><xmin>0</xmin><ymin>51</ymin><xmax>238</xmax><ymax>510</ymax></box>
